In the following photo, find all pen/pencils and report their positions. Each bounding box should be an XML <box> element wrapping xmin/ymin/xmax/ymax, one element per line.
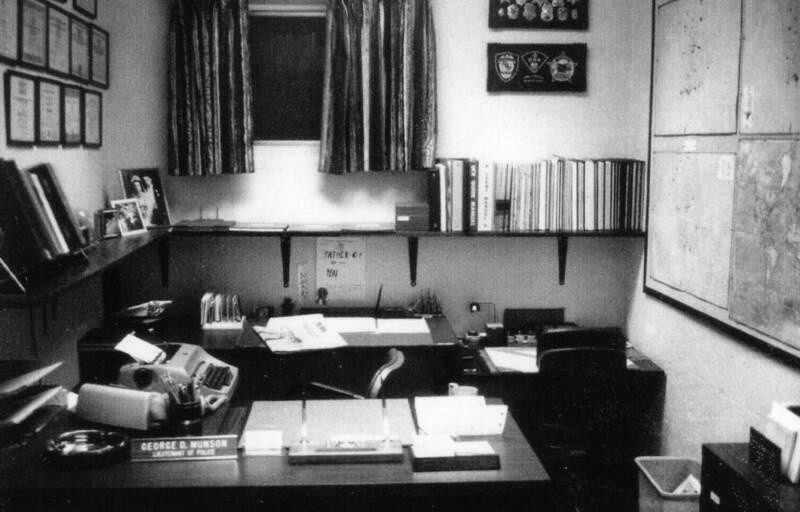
<box><xmin>159</xmin><ymin>370</ymin><xmax>195</xmax><ymax>404</ymax></box>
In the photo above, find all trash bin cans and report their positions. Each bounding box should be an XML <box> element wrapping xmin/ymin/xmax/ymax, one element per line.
<box><xmin>634</xmin><ymin>455</ymin><xmax>702</xmax><ymax>512</ymax></box>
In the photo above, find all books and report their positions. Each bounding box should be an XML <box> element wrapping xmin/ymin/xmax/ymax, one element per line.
<box><xmin>287</xmin><ymin>432</ymin><xmax>405</xmax><ymax>464</ymax></box>
<box><xmin>409</xmin><ymin>441</ymin><xmax>501</xmax><ymax>483</ymax></box>
<box><xmin>748</xmin><ymin>416</ymin><xmax>798</xmax><ymax>480</ymax></box>
<box><xmin>768</xmin><ymin>402</ymin><xmax>800</xmax><ymax>486</ymax></box>
<box><xmin>671</xmin><ymin>473</ymin><xmax>703</xmax><ymax>496</ymax></box>
<box><xmin>427</xmin><ymin>156</ymin><xmax>646</xmax><ymax>234</ymax></box>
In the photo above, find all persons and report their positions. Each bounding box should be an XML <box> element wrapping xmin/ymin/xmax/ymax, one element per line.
<box><xmin>142</xmin><ymin>172</ymin><xmax>167</xmax><ymax>226</ymax></box>
<box><xmin>131</xmin><ymin>175</ymin><xmax>149</xmax><ymax>221</ymax></box>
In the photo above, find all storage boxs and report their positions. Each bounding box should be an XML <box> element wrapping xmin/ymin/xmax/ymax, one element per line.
<box><xmin>392</xmin><ymin>200</ymin><xmax>431</xmax><ymax>232</ymax></box>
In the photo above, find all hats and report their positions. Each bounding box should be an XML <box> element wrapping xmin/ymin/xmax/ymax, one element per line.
<box><xmin>130</xmin><ymin>175</ymin><xmax>141</xmax><ymax>183</ymax></box>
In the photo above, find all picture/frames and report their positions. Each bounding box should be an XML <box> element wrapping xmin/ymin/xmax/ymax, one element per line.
<box><xmin>99</xmin><ymin>167</ymin><xmax>171</xmax><ymax>239</ymax></box>
<box><xmin>0</xmin><ymin>1</ymin><xmax>111</xmax><ymax>149</ymax></box>
